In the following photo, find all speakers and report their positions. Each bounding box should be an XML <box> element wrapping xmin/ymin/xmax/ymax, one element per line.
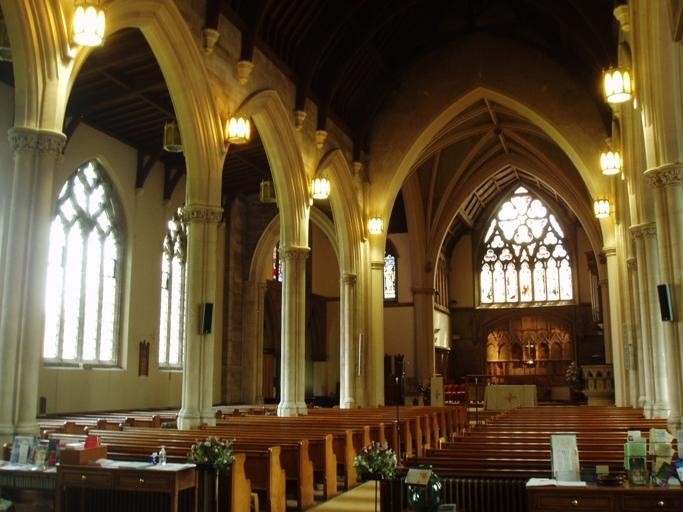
<box><xmin>657</xmin><ymin>283</ymin><xmax>674</xmax><ymax>322</ymax></box>
<box><xmin>199</xmin><ymin>302</ymin><xmax>213</xmax><ymax>336</ymax></box>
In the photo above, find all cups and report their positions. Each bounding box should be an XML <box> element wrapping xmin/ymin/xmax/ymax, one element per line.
<box><xmin>84</xmin><ymin>434</ymin><xmax>99</xmax><ymax>449</ymax></box>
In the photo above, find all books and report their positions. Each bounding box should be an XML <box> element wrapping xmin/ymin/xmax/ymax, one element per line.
<box><xmin>0</xmin><ymin>433</ymin><xmax>101</xmax><ymax>473</ymax></box>
<box><xmin>622</xmin><ymin>428</ymin><xmax>682</xmax><ymax>489</ymax></box>
<box><xmin>549</xmin><ymin>433</ymin><xmax>580</xmax><ymax>481</ymax></box>
<box><xmin>523</xmin><ymin>477</ymin><xmax>588</xmax><ymax>489</ymax></box>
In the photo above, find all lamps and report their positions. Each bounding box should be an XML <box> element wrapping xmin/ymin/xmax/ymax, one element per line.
<box><xmin>224</xmin><ymin>113</ymin><xmax>253</xmax><ymax>146</ymax></box>
<box><xmin>70</xmin><ymin>0</ymin><xmax>107</xmax><ymax>47</ymax></box>
<box><xmin>310</xmin><ymin>176</ymin><xmax>332</xmax><ymax>200</ymax></box>
<box><xmin>366</xmin><ymin>215</ymin><xmax>383</xmax><ymax>236</ymax></box>
<box><xmin>592</xmin><ymin>62</ymin><xmax>637</xmax><ymax>219</ymax></box>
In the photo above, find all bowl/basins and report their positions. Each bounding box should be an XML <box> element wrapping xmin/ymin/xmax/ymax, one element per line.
<box><xmin>593</xmin><ymin>473</ymin><xmax>627</xmax><ymax>487</ymax></box>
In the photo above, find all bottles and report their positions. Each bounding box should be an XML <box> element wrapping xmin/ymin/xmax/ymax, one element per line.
<box><xmin>18</xmin><ymin>437</ymin><xmax>57</xmax><ymax>471</ymax></box>
<box><xmin>152</xmin><ymin>446</ymin><xmax>167</xmax><ymax>465</ymax></box>
